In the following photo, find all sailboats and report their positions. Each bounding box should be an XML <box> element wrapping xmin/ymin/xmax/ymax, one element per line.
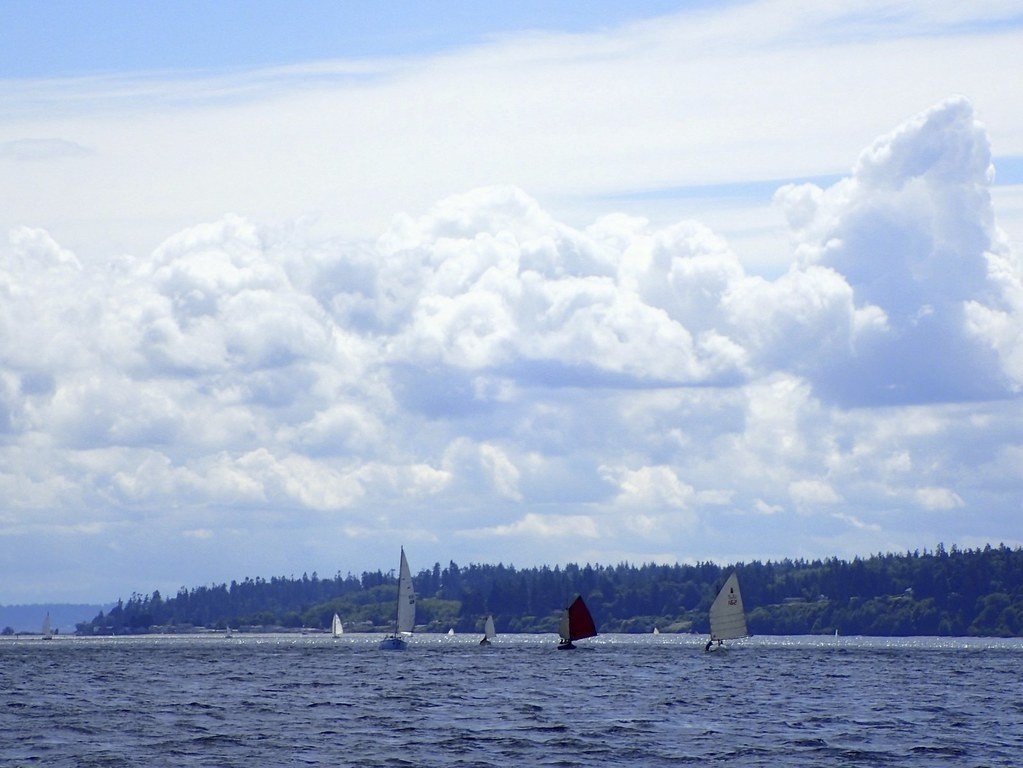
<box><xmin>652</xmin><ymin>627</ymin><xmax>660</xmax><ymax>635</ymax></box>
<box><xmin>703</xmin><ymin>571</ymin><xmax>751</xmax><ymax>655</ymax></box>
<box><xmin>480</xmin><ymin>616</ymin><xmax>497</xmax><ymax>646</ymax></box>
<box><xmin>378</xmin><ymin>544</ymin><xmax>417</xmax><ymax>649</ymax></box>
<box><xmin>556</xmin><ymin>596</ymin><xmax>600</xmax><ymax>650</ymax></box>
<box><xmin>42</xmin><ymin>611</ymin><xmax>53</xmax><ymax>640</ymax></box>
<box><xmin>447</xmin><ymin>628</ymin><xmax>457</xmax><ymax>638</ymax></box>
<box><xmin>225</xmin><ymin>626</ymin><xmax>233</xmax><ymax>638</ymax></box>
<box><xmin>331</xmin><ymin>611</ymin><xmax>344</xmax><ymax>638</ymax></box>
